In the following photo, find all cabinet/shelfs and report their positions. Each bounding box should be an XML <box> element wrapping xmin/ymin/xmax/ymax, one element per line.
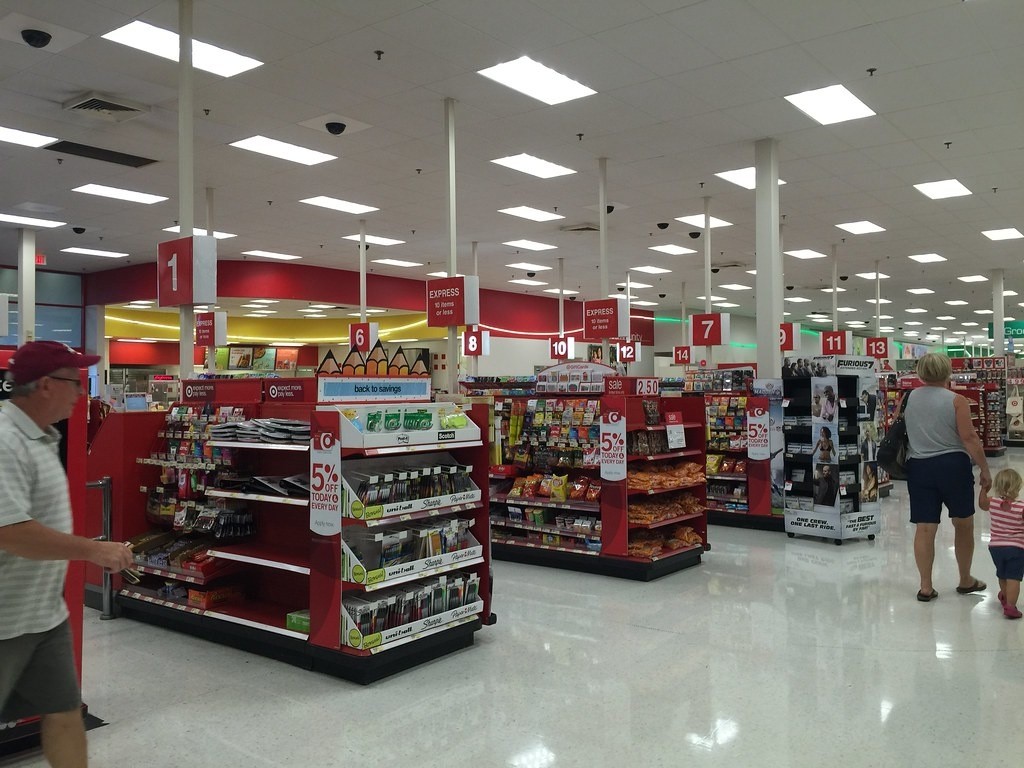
<box><xmin>783</xmin><ymin>357</ymin><xmax>883</xmax><ymax>542</ymax></box>
<box><xmin>701</xmin><ymin>390</ymin><xmax>788</xmax><ymax>530</ymax></box>
<box><xmin>463</xmin><ymin>380</ymin><xmax>711</xmax><ymax>583</ymax></box>
<box><xmin>87</xmin><ymin>380</ymin><xmax>493</xmax><ymax>687</ymax></box>
<box><xmin>878</xmin><ymin>354</ymin><xmax>1024</xmax><ymax>458</ymax></box>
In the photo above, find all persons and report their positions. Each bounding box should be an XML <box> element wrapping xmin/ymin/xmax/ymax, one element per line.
<box><xmin>883</xmin><ymin>360</ymin><xmax>893</xmax><ymax>370</ymax></box>
<box><xmin>782</xmin><ymin>358</ymin><xmax>826</xmax><ymax>377</ymax></box>
<box><xmin>813</xmin><ymin>386</ymin><xmax>836</xmax><ymax>422</ymax></box>
<box><xmin>863</xmin><ymin>462</ymin><xmax>878</xmax><ymax>502</ymax></box>
<box><xmin>894</xmin><ymin>353</ymin><xmax>993</xmax><ymax>602</ymax></box>
<box><xmin>979</xmin><ymin>469</ymin><xmax>1024</xmax><ymax>618</ymax></box>
<box><xmin>815</xmin><ymin>467</ymin><xmax>836</xmax><ymax>507</ymax></box>
<box><xmin>813</xmin><ymin>426</ymin><xmax>836</xmax><ymax>463</ymax></box>
<box><xmin>861</xmin><ymin>390</ymin><xmax>877</xmax><ymax>420</ymax></box>
<box><xmin>861</xmin><ymin>428</ymin><xmax>877</xmax><ymax>461</ymax></box>
<box><xmin>592</xmin><ymin>347</ymin><xmax>601</xmax><ymax>363</ymax></box>
<box><xmin>0</xmin><ymin>340</ymin><xmax>133</xmax><ymax>768</ymax></box>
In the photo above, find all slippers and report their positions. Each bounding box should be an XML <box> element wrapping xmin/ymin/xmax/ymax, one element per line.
<box><xmin>956</xmin><ymin>579</ymin><xmax>987</xmax><ymax>593</ymax></box>
<box><xmin>917</xmin><ymin>587</ymin><xmax>938</xmax><ymax>602</ymax></box>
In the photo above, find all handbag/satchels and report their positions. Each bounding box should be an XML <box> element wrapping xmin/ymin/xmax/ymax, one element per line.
<box><xmin>1009</xmin><ymin>415</ymin><xmax>1024</xmax><ymax>432</ymax></box>
<box><xmin>1006</xmin><ymin>385</ymin><xmax>1023</xmax><ymax>417</ymax></box>
<box><xmin>877</xmin><ymin>387</ymin><xmax>913</xmax><ymax>481</ymax></box>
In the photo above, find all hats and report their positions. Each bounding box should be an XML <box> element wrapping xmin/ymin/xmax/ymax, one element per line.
<box><xmin>6</xmin><ymin>339</ymin><xmax>102</xmax><ymax>385</ymax></box>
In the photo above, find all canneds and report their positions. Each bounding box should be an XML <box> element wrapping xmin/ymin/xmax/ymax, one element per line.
<box><xmin>525</xmin><ymin>507</ymin><xmax>548</xmax><ymax>526</ymax></box>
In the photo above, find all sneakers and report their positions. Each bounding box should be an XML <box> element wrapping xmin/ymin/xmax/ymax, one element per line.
<box><xmin>1003</xmin><ymin>605</ymin><xmax>1022</xmax><ymax>618</ymax></box>
<box><xmin>998</xmin><ymin>591</ymin><xmax>1009</xmax><ymax>608</ymax></box>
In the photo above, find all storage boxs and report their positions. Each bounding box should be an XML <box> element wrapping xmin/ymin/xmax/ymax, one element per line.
<box><xmin>338</xmin><ymin>458</ymin><xmax>484</xmax><ymax>650</ymax></box>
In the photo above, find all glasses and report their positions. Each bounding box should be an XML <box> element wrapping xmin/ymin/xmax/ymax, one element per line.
<box><xmin>48</xmin><ymin>375</ymin><xmax>81</xmax><ymax>386</ymax></box>
